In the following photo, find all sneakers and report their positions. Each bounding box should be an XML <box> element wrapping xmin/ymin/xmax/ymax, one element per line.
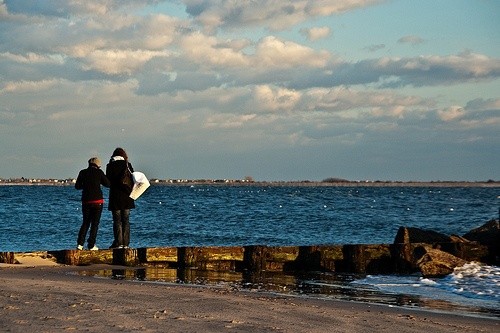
<box><xmin>88</xmin><ymin>245</ymin><xmax>99</xmax><ymax>250</ymax></box>
<box><xmin>76</xmin><ymin>244</ymin><xmax>84</xmax><ymax>250</ymax></box>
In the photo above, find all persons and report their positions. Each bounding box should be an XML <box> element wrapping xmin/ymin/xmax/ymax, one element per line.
<box><xmin>106</xmin><ymin>147</ymin><xmax>138</xmax><ymax>250</ymax></box>
<box><xmin>73</xmin><ymin>156</ymin><xmax>109</xmax><ymax>250</ymax></box>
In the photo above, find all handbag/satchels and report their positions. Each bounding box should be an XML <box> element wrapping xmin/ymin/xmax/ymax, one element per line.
<box><xmin>130</xmin><ymin>172</ymin><xmax>150</xmax><ymax>200</ymax></box>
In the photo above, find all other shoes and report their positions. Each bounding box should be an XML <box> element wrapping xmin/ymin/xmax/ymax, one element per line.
<box><xmin>110</xmin><ymin>244</ymin><xmax>132</xmax><ymax>249</ymax></box>
